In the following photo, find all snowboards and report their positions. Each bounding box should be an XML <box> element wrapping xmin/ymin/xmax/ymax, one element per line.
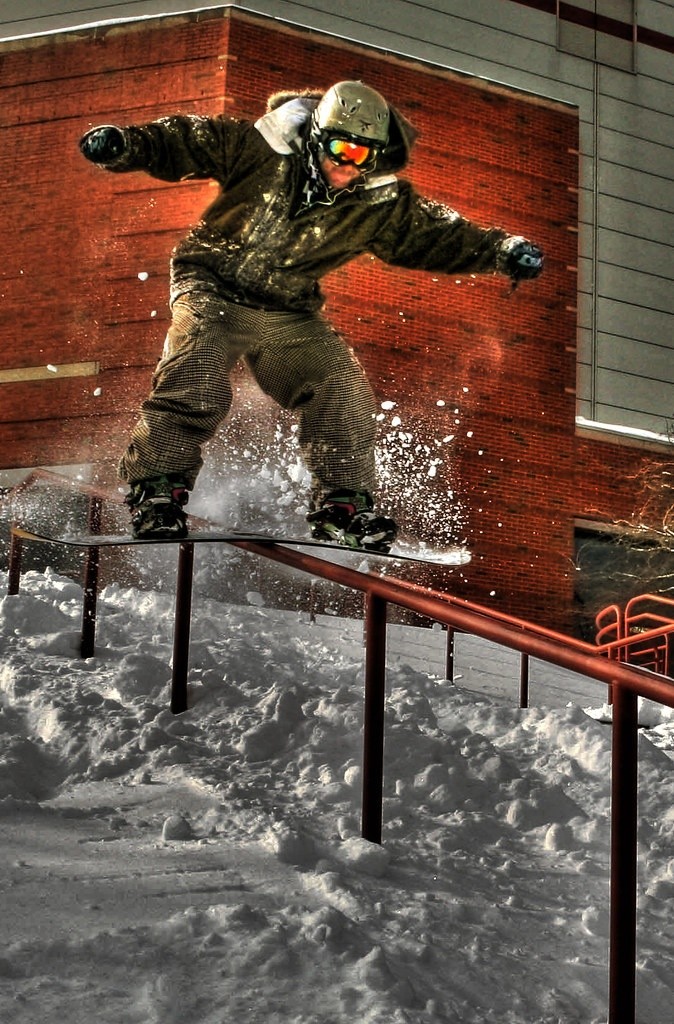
<box><xmin>9</xmin><ymin>520</ymin><xmax>471</xmax><ymax>569</ymax></box>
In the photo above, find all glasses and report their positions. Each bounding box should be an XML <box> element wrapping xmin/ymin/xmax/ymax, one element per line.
<box><xmin>319</xmin><ymin>129</ymin><xmax>386</xmax><ymax>169</ymax></box>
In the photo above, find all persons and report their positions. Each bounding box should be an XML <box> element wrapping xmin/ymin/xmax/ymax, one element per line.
<box><xmin>77</xmin><ymin>79</ymin><xmax>548</xmax><ymax>550</ymax></box>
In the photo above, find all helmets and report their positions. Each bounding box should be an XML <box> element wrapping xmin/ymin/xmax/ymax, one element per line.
<box><xmin>309</xmin><ymin>80</ymin><xmax>390</xmax><ymax>172</ymax></box>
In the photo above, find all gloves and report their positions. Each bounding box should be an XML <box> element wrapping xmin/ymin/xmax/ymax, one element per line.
<box><xmin>79</xmin><ymin>126</ymin><xmax>125</xmax><ymax>164</ymax></box>
<box><xmin>504</xmin><ymin>242</ymin><xmax>544</xmax><ymax>280</ymax></box>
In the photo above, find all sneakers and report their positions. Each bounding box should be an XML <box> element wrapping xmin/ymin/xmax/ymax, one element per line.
<box><xmin>306</xmin><ymin>480</ymin><xmax>398</xmax><ymax>554</ymax></box>
<box><xmin>122</xmin><ymin>474</ymin><xmax>190</xmax><ymax>540</ymax></box>
